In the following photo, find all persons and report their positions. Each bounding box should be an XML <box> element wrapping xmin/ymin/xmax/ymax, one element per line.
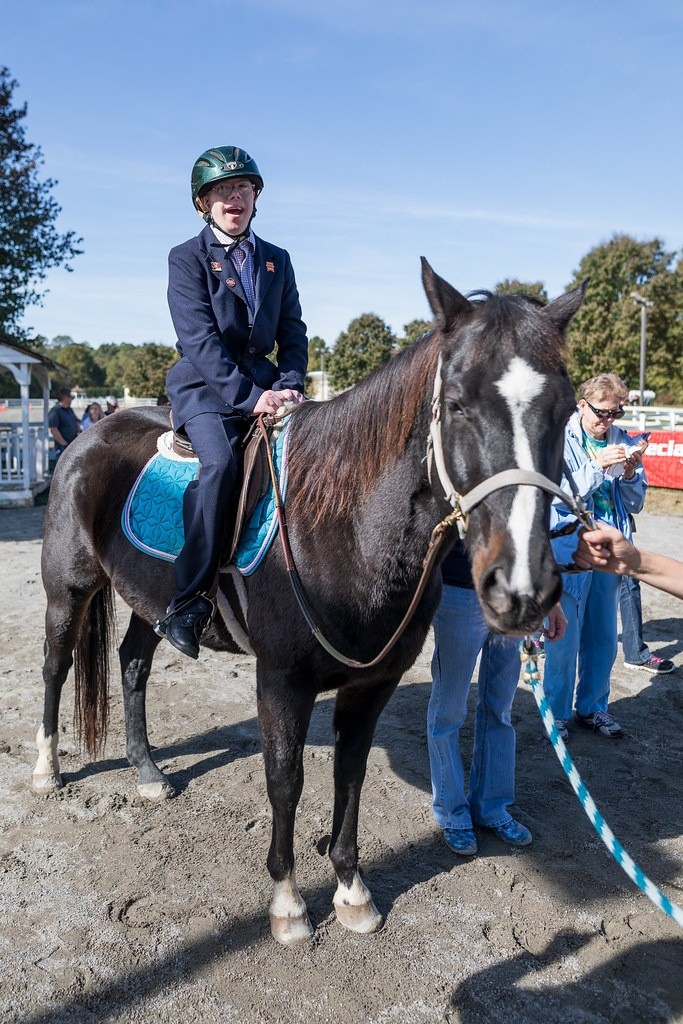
<box><xmin>426</xmin><ymin>540</ymin><xmax>564</xmax><ymax>856</ymax></box>
<box><xmin>48</xmin><ymin>388</ymin><xmax>78</xmax><ymax>455</ymax></box>
<box><xmin>82</xmin><ymin>396</ymin><xmax>118</xmax><ymax>431</ymax></box>
<box><xmin>163</xmin><ymin>145</ymin><xmax>309</xmax><ymax>659</ymax></box>
<box><xmin>567</xmin><ymin>372</ymin><xmax>674</xmax><ymax>674</ymax></box>
<box><xmin>571</xmin><ymin>520</ymin><xmax>683</xmax><ymax>600</ymax></box>
<box><xmin>541</xmin><ymin>425</ymin><xmax>628</xmax><ymax>743</ymax></box>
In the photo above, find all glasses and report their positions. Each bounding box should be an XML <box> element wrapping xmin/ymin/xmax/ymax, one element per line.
<box><xmin>202</xmin><ymin>179</ymin><xmax>256</xmax><ymax>200</ymax></box>
<box><xmin>581</xmin><ymin>397</ymin><xmax>626</xmax><ymax>418</ymax></box>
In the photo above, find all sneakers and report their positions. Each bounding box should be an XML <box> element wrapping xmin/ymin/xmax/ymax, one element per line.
<box><xmin>575</xmin><ymin>710</ymin><xmax>622</xmax><ymax>738</ymax></box>
<box><xmin>531</xmin><ymin>638</ymin><xmax>547</xmax><ymax>659</ymax></box>
<box><xmin>542</xmin><ymin>717</ymin><xmax>569</xmax><ymax>744</ymax></box>
<box><xmin>624</xmin><ymin>655</ymin><xmax>675</xmax><ymax>674</ymax></box>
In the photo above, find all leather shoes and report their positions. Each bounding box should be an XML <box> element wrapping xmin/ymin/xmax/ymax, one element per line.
<box><xmin>166</xmin><ymin>602</ymin><xmax>212</xmax><ymax>660</ymax></box>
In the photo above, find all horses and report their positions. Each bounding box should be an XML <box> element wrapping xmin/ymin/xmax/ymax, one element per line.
<box><xmin>30</xmin><ymin>254</ymin><xmax>593</xmax><ymax>948</ymax></box>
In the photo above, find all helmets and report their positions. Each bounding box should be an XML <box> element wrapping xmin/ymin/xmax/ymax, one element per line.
<box><xmin>191</xmin><ymin>147</ymin><xmax>264</xmax><ymax>210</ymax></box>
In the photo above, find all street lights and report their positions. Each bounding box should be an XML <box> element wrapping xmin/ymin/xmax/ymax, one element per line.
<box><xmin>314</xmin><ymin>345</ymin><xmax>329</xmax><ymax>399</ymax></box>
<box><xmin>631</xmin><ymin>292</ymin><xmax>655</xmax><ymax>406</ymax></box>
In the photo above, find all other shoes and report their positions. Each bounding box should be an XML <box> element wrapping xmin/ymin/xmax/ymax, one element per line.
<box><xmin>494</xmin><ymin>820</ymin><xmax>532</xmax><ymax>847</ymax></box>
<box><xmin>443</xmin><ymin>828</ymin><xmax>478</xmax><ymax>854</ymax></box>
